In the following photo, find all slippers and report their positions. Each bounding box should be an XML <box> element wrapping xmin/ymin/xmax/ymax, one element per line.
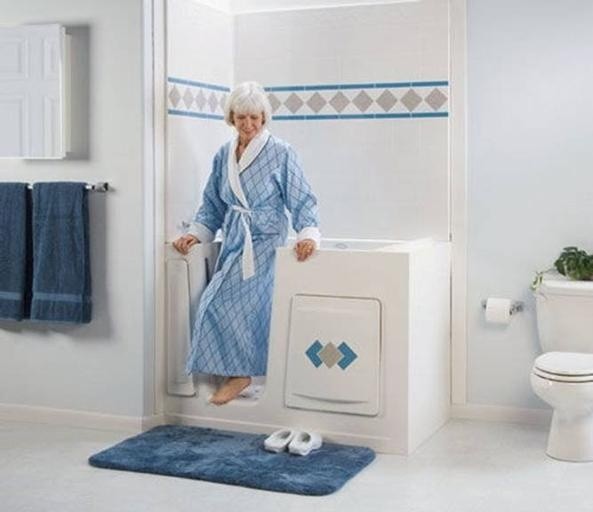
<box><xmin>289</xmin><ymin>432</ymin><xmax>322</xmax><ymax>456</ymax></box>
<box><xmin>264</xmin><ymin>429</ymin><xmax>295</xmax><ymax>453</ymax></box>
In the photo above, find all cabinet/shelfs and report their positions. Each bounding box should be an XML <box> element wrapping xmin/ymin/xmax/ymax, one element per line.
<box><xmin>0</xmin><ymin>22</ymin><xmax>86</xmax><ymax>162</ymax></box>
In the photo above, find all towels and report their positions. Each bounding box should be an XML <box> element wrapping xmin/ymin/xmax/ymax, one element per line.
<box><xmin>0</xmin><ymin>181</ymin><xmax>93</xmax><ymax>326</ymax></box>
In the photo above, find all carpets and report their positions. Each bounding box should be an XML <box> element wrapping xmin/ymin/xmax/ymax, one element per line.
<box><xmin>88</xmin><ymin>421</ymin><xmax>376</xmax><ymax>497</ymax></box>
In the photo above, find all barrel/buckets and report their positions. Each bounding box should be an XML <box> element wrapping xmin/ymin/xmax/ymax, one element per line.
<box><xmin>484</xmin><ymin>297</ymin><xmax>515</xmax><ymax>322</ymax></box>
<box><xmin>531</xmin><ymin>273</ymin><xmax>592</xmax><ymax>462</ymax></box>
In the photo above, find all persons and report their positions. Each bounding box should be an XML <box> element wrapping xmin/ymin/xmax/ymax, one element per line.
<box><xmin>174</xmin><ymin>79</ymin><xmax>322</xmax><ymax>407</ymax></box>
